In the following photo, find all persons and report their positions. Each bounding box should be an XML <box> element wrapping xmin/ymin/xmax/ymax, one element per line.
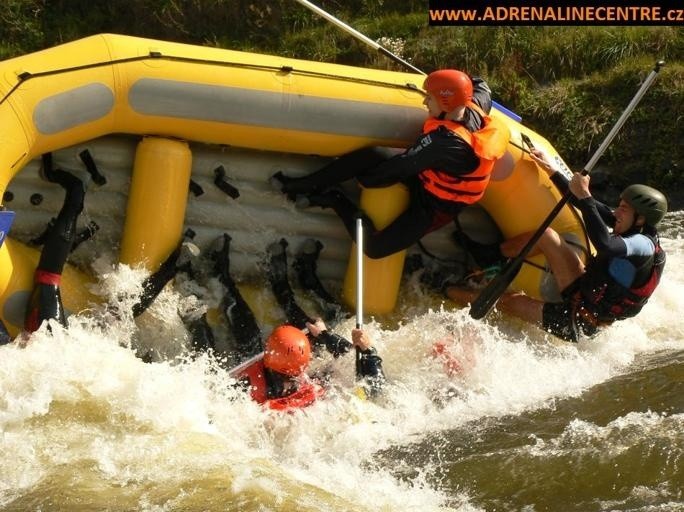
<box><xmin>173</xmin><ymin>228</ymin><xmax>325</xmax><ymax>416</ymax></box>
<box><xmin>260</xmin><ymin>235</ymin><xmax>477</xmax><ymax>414</ymax></box>
<box><xmin>262</xmin><ymin>66</ymin><xmax>509</xmax><ymax>262</ymax></box>
<box><xmin>21</xmin><ymin>166</ymin><xmax>203</xmax><ymax>349</ymax></box>
<box><xmin>410</xmin><ymin>142</ymin><xmax>668</xmax><ymax>345</ymax></box>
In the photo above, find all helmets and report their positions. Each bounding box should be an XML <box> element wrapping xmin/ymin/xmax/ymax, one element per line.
<box><xmin>618</xmin><ymin>183</ymin><xmax>669</xmax><ymax>229</ymax></box>
<box><xmin>259</xmin><ymin>325</ymin><xmax>311</xmax><ymax>379</ymax></box>
<box><xmin>419</xmin><ymin>68</ymin><xmax>475</xmax><ymax>120</ymax></box>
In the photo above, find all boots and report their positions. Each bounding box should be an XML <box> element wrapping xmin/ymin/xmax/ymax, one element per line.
<box><xmin>404</xmin><ymin>227</ymin><xmax>507</xmax><ymax>299</ymax></box>
<box><xmin>159</xmin><ymin>167</ymin><xmax>344</xmax><ymax>295</ymax></box>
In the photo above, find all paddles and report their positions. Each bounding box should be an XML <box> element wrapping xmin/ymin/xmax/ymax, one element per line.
<box><xmin>470</xmin><ymin>59</ymin><xmax>667</xmax><ymax>318</ymax></box>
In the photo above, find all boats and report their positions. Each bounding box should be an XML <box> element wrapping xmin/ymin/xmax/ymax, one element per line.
<box><xmin>2</xmin><ymin>34</ymin><xmax>602</xmax><ymax>411</ymax></box>
